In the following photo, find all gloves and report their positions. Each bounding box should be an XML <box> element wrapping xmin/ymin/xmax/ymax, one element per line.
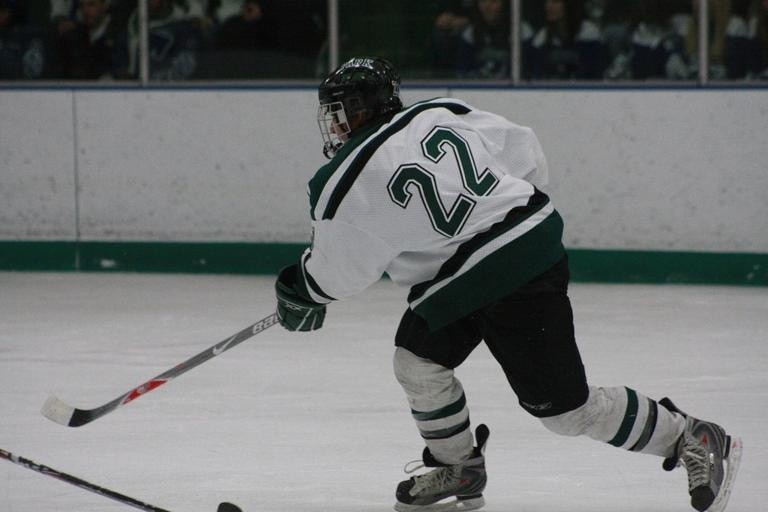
<box><xmin>274</xmin><ymin>264</ymin><xmax>327</xmax><ymax>332</ymax></box>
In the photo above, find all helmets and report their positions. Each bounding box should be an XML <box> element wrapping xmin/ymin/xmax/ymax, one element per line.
<box><xmin>316</xmin><ymin>56</ymin><xmax>401</xmax><ymax>159</ymax></box>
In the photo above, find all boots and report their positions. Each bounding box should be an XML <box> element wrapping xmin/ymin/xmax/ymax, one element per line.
<box><xmin>658</xmin><ymin>397</ymin><xmax>726</xmax><ymax>511</ymax></box>
<box><xmin>396</xmin><ymin>423</ymin><xmax>490</xmax><ymax>507</ymax></box>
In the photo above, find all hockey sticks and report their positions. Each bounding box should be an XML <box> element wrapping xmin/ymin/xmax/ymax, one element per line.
<box><xmin>0</xmin><ymin>448</ymin><xmax>240</xmax><ymax>512</ymax></box>
<box><xmin>40</xmin><ymin>310</ymin><xmax>283</xmax><ymax>426</ymax></box>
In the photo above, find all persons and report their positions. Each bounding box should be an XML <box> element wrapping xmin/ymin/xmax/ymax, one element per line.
<box><xmin>0</xmin><ymin>1</ymin><xmax>767</xmax><ymax>87</ymax></box>
<box><xmin>275</xmin><ymin>57</ymin><xmax>728</xmax><ymax>512</ymax></box>
